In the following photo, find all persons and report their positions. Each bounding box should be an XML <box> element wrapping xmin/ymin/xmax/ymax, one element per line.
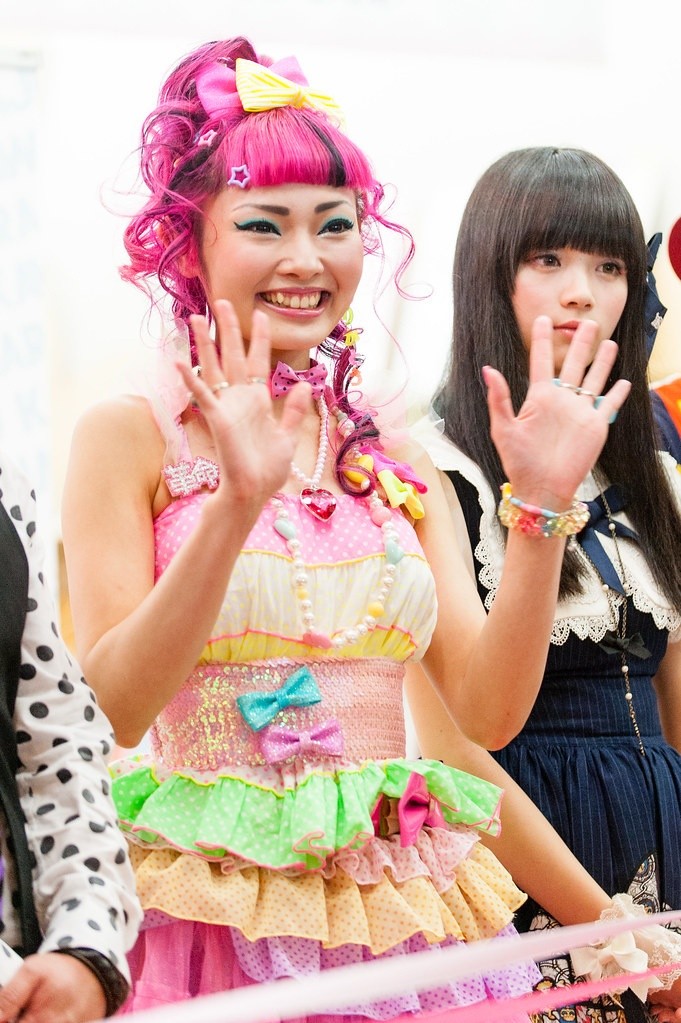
<box><xmin>0</xmin><ymin>450</ymin><xmax>144</xmax><ymax>1023</ymax></box>
<box><xmin>60</xmin><ymin>38</ymin><xmax>631</xmax><ymax>1023</ymax></box>
<box><xmin>408</xmin><ymin>147</ymin><xmax>681</xmax><ymax>1022</ymax></box>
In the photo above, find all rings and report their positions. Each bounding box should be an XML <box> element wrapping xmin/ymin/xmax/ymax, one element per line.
<box><xmin>578</xmin><ymin>389</ymin><xmax>597</xmax><ymax>400</ymax></box>
<box><xmin>210</xmin><ymin>380</ymin><xmax>229</xmax><ymax>393</ymax></box>
<box><xmin>249</xmin><ymin>376</ymin><xmax>267</xmax><ymax>384</ymax></box>
<box><xmin>559</xmin><ymin>379</ymin><xmax>578</xmax><ymax>394</ymax></box>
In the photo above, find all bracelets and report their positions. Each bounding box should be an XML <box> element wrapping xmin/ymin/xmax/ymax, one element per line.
<box><xmin>498</xmin><ymin>501</ymin><xmax>591</xmax><ymax>541</ymax></box>
<box><xmin>501</xmin><ymin>483</ymin><xmax>588</xmax><ymax>518</ymax></box>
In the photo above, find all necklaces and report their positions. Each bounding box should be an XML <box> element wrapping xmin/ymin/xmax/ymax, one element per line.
<box><xmin>272</xmin><ymin>403</ymin><xmax>403</xmax><ymax>649</ymax></box>
<box><xmin>291</xmin><ymin>393</ymin><xmax>338</xmax><ymax>523</ymax></box>
<box><xmin>574</xmin><ymin>466</ymin><xmax>644</xmax><ymax>758</ymax></box>
